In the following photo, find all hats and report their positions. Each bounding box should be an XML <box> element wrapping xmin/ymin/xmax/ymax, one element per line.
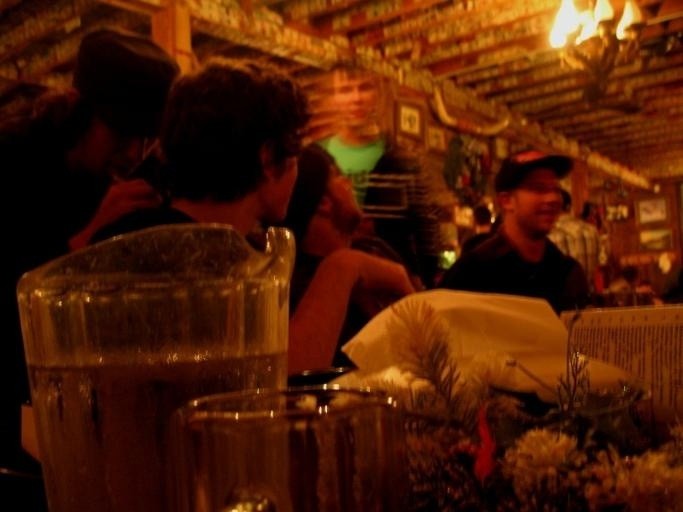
<box><xmin>72</xmin><ymin>28</ymin><xmax>179</xmax><ymax>117</ymax></box>
<box><xmin>494</xmin><ymin>147</ymin><xmax>574</xmax><ymax>190</ymax></box>
<box><xmin>260</xmin><ymin>143</ymin><xmax>332</xmax><ymax>230</ymax></box>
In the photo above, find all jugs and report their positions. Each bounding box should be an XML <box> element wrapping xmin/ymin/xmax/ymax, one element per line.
<box><xmin>18</xmin><ymin>223</ymin><xmax>298</xmax><ymax>512</ymax></box>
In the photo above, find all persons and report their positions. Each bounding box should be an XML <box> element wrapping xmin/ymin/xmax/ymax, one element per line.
<box><xmin>441</xmin><ymin>146</ymin><xmax>591</xmax><ymax>311</ymax></box>
<box><xmin>2</xmin><ymin>27</ymin><xmax>416</xmax><ymax>406</ymax></box>
<box><xmin>613</xmin><ymin>265</ymin><xmax>638</xmax><ymax>307</ymax></box>
<box><xmin>549</xmin><ymin>190</ymin><xmax>607</xmax><ymax>279</ymax></box>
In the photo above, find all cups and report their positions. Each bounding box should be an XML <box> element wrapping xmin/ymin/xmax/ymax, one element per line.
<box><xmin>183</xmin><ymin>383</ymin><xmax>396</xmax><ymax>511</ymax></box>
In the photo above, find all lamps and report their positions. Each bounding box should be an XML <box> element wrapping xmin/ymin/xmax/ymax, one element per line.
<box><xmin>548</xmin><ymin>0</ymin><xmax>647</xmax><ymax>102</ymax></box>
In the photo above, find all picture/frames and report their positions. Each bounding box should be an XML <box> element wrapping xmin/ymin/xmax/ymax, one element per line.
<box><xmin>395</xmin><ymin>100</ymin><xmax>425</xmax><ymax>144</ymax></box>
<box><xmin>635</xmin><ymin>197</ymin><xmax>672</xmax><ymax>227</ymax></box>
<box><xmin>637</xmin><ymin>229</ymin><xmax>674</xmax><ymax>252</ymax></box>
<box><xmin>427</xmin><ymin>123</ymin><xmax>449</xmax><ymax>156</ymax></box>
